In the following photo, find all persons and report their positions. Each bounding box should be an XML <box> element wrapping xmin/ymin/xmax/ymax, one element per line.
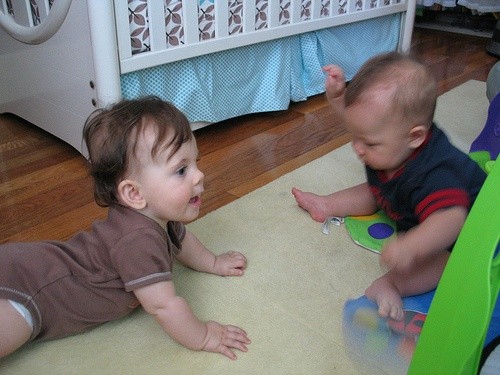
<box><xmin>0</xmin><ymin>95</ymin><xmax>251</xmax><ymax>360</ymax></box>
<box><xmin>292</xmin><ymin>50</ymin><xmax>500</xmax><ymax>321</ymax></box>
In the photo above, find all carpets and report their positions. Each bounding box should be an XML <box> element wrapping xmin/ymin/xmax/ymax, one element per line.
<box><xmin>0</xmin><ymin>79</ymin><xmax>490</xmax><ymax>374</ymax></box>
